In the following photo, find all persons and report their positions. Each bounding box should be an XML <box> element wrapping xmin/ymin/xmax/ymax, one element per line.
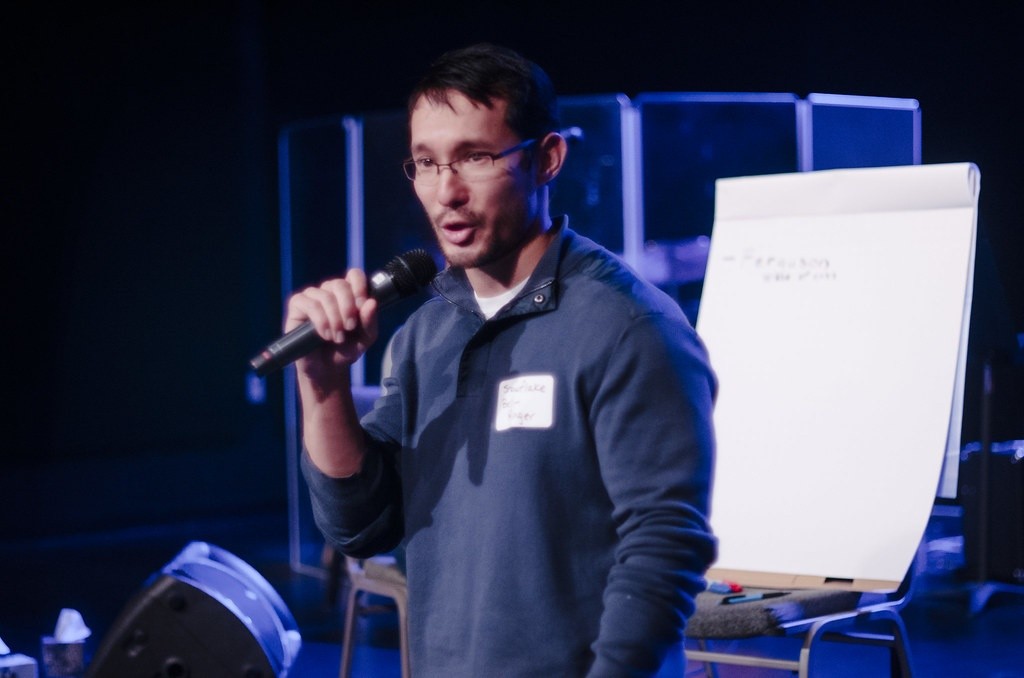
<box><xmin>284</xmin><ymin>49</ymin><xmax>719</xmax><ymax>677</ymax></box>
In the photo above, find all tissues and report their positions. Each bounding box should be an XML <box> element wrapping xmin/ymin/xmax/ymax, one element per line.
<box><xmin>40</xmin><ymin>608</ymin><xmax>92</xmax><ymax>675</ymax></box>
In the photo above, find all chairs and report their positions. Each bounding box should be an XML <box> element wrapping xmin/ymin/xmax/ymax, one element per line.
<box><xmin>683</xmin><ymin>552</ymin><xmax>919</xmax><ymax>678</ymax></box>
<box><xmin>339</xmin><ymin>386</ymin><xmax>409</xmax><ymax>678</ymax></box>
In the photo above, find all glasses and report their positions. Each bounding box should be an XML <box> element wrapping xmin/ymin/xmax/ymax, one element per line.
<box><xmin>401</xmin><ymin>137</ymin><xmax>542</xmax><ymax>184</ymax></box>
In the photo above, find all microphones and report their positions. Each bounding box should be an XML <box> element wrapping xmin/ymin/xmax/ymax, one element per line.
<box><xmin>250</xmin><ymin>248</ymin><xmax>438</xmax><ymax>377</ymax></box>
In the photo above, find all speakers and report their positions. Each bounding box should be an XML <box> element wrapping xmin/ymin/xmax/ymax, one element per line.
<box><xmin>82</xmin><ymin>539</ymin><xmax>303</xmax><ymax>678</ymax></box>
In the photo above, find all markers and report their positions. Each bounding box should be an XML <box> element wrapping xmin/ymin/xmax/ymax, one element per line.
<box><xmin>707</xmin><ymin>581</ymin><xmax>731</xmax><ymax>595</ymax></box>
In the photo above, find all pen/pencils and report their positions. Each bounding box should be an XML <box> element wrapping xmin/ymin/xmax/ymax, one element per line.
<box><xmin>719</xmin><ymin>590</ymin><xmax>793</xmax><ymax>606</ymax></box>
